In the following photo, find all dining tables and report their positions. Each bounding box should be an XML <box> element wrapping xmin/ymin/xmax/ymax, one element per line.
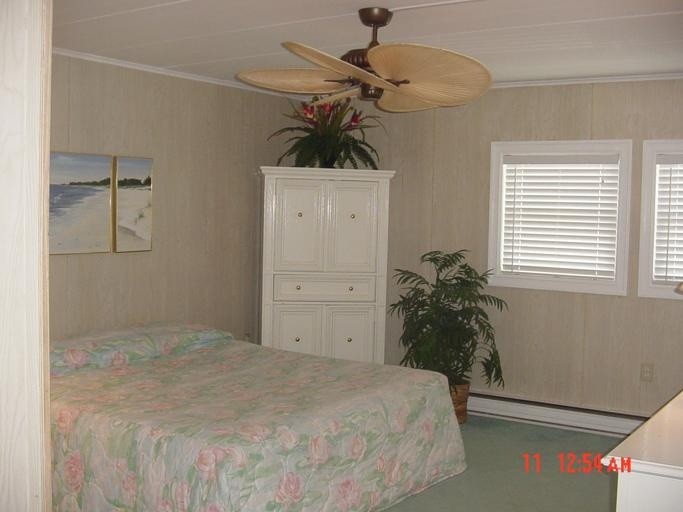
<box><xmin>386</xmin><ymin>251</ymin><xmax>509</xmax><ymax>424</ymax></box>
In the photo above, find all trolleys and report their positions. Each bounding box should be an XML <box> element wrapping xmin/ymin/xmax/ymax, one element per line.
<box><xmin>235</xmin><ymin>7</ymin><xmax>493</xmax><ymax>113</ymax></box>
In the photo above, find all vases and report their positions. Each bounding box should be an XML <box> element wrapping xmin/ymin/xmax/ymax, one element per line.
<box><xmin>266</xmin><ymin>96</ymin><xmax>388</xmax><ymax>171</ymax></box>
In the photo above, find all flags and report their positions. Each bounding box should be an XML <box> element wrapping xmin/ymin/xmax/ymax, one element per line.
<box><xmin>319</xmin><ymin>152</ymin><xmax>339</xmax><ymax>168</ymax></box>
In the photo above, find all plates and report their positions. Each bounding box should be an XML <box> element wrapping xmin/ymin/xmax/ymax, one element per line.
<box><xmin>256</xmin><ymin>166</ymin><xmax>396</xmax><ymax>364</ymax></box>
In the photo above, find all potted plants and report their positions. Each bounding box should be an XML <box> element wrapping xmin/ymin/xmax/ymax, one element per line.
<box><xmin>113</xmin><ymin>156</ymin><xmax>154</xmax><ymax>253</ymax></box>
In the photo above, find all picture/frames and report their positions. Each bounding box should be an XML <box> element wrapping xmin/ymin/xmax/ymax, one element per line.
<box><xmin>600</xmin><ymin>391</ymin><xmax>683</xmax><ymax>511</ymax></box>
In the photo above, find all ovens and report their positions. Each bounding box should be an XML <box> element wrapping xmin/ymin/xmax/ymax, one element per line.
<box><xmin>50</xmin><ymin>323</ymin><xmax>468</xmax><ymax>512</ymax></box>
